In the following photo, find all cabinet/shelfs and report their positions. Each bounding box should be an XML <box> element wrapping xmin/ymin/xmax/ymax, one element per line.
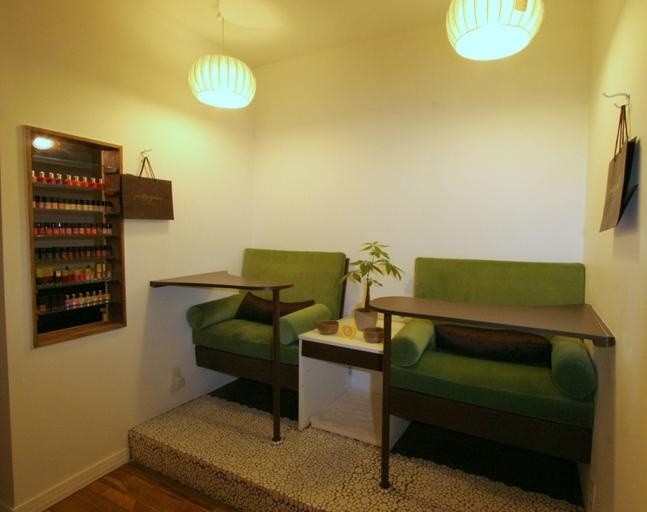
<box><xmin>25</xmin><ymin>124</ymin><xmax>126</xmax><ymax>348</ymax></box>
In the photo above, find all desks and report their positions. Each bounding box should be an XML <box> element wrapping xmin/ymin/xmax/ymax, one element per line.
<box><xmin>150</xmin><ymin>271</ymin><xmax>293</xmax><ymax>446</ymax></box>
<box><xmin>368</xmin><ymin>296</ymin><xmax>615</xmax><ymax>489</ymax></box>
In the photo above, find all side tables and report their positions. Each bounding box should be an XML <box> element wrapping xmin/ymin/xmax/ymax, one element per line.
<box><xmin>298</xmin><ymin>317</ymin><xmax>411</xmax><ymax>451</ymax></box>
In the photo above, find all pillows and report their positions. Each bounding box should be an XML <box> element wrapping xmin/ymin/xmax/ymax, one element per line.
<box><xmin>434</xmin><ymin>324</ymin><xmax>552</xmax><ymax>365</ymax></box>
<box><xmin>233</xmin><ymin>291</ymin><xmax>314</xmax><ymax>325</ymax></box>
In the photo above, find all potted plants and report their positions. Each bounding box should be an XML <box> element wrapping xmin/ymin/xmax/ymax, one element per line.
<box><xmin>336</xmin><ymin>241</ymin><xmax>406</xmax><ymax>331</ymax></box>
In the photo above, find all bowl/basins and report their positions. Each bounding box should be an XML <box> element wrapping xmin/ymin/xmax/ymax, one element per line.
<box><xmin>364</xmin><ymin>327</ymin><xmax>384</xmax><ymax>343</ymax></box>
<box><xmin>318</xmin><ymin>321</ymin><xmax>339</xmax><ymax>336</ymax></box>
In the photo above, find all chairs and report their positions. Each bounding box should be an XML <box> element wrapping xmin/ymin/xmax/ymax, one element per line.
<box><xmin>390</xmin><ymin>257</ymin><xmax>596</xmax><ymax>464</ymax></box>
<box><xmin>186</xmin><ymin>248</ymin><xmax>346</xmax><ymax>392</ymax></box>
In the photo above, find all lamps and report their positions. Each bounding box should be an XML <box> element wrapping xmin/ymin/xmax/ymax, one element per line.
<box><xmin>446</xmin><ymin>0</ymin><xmax>545</xmax><ymax>62</ymax></box>
<box><xmin>187</xmin><ymin>18</ymin><xmax>257</xmax><ymax>109</ymax></box>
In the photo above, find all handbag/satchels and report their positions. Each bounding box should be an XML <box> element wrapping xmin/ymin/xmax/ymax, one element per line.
<box><xmin>599</xmin><ymin>105</ymin><xmax>638</xmax><ymax>232</ymax></box>
<box><xmin>121</xmin><ymin>157</ymin><xmax>173</xmax><ymax>219</ymax></box>
